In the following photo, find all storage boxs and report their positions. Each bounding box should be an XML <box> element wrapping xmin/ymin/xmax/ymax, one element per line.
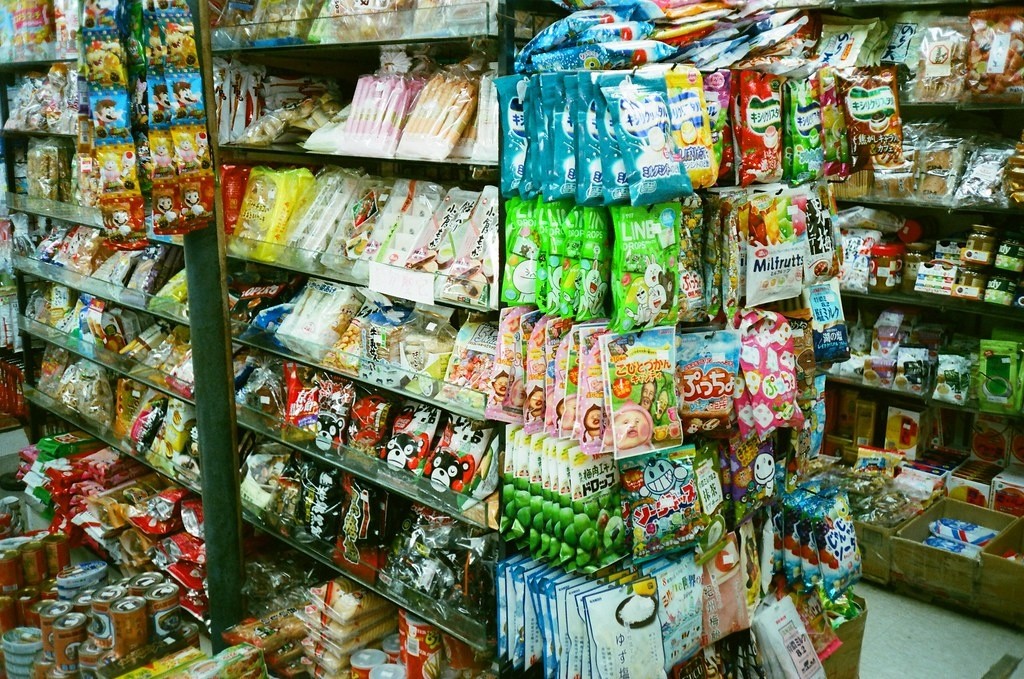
<box><xmin>821</xmin><ymin>592</ymin><xmax>867</xmax><ymax>679</ymax></box>
<box><xmin>890</xmin><ymin>497</ymin><xmax>1018</xmax><ymax>609</ymax></box>
<box><xmin>980</xmin><ymin>516</ymin><xmax>1023</xmax><ymax>629</ymax></box>
<box><xmin>853</xmin><ymin>502</ymin><xmax>930</xmax><ymax>584</ymax></box>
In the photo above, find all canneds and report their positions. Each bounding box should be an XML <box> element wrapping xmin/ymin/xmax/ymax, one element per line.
<box><xmin>0</xmin><ymin>535</ymin><xmax>200</xmax><ymax>679</ymax></box>
<box><xmin>870</xmin><ymin>215</ymin><xmax>1024</xmax><ymax>306</ymax></box>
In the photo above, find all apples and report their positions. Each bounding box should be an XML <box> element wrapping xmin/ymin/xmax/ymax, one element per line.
<box><xmin>504</xmin><ymin>475</ymin><xmax>623</xmax><ymax>571</ymax></box>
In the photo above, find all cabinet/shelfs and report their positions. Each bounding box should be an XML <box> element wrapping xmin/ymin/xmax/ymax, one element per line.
<box><xmin>1</xmin><ymin>0</ymin><xmax>1024</xmax><ymax>679</ymax></box>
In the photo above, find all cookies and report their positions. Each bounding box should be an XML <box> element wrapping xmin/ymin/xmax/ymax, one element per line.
<box><xmin>232</xmin><ymin>577</ymin><xmax>398</xmax><ymax>679</ymax></box>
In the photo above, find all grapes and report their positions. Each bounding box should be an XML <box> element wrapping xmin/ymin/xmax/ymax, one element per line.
<box><xmin>776</xmin><ymin>506</ymin><xmax>829</xmax><ymax>550</ymax></box>
